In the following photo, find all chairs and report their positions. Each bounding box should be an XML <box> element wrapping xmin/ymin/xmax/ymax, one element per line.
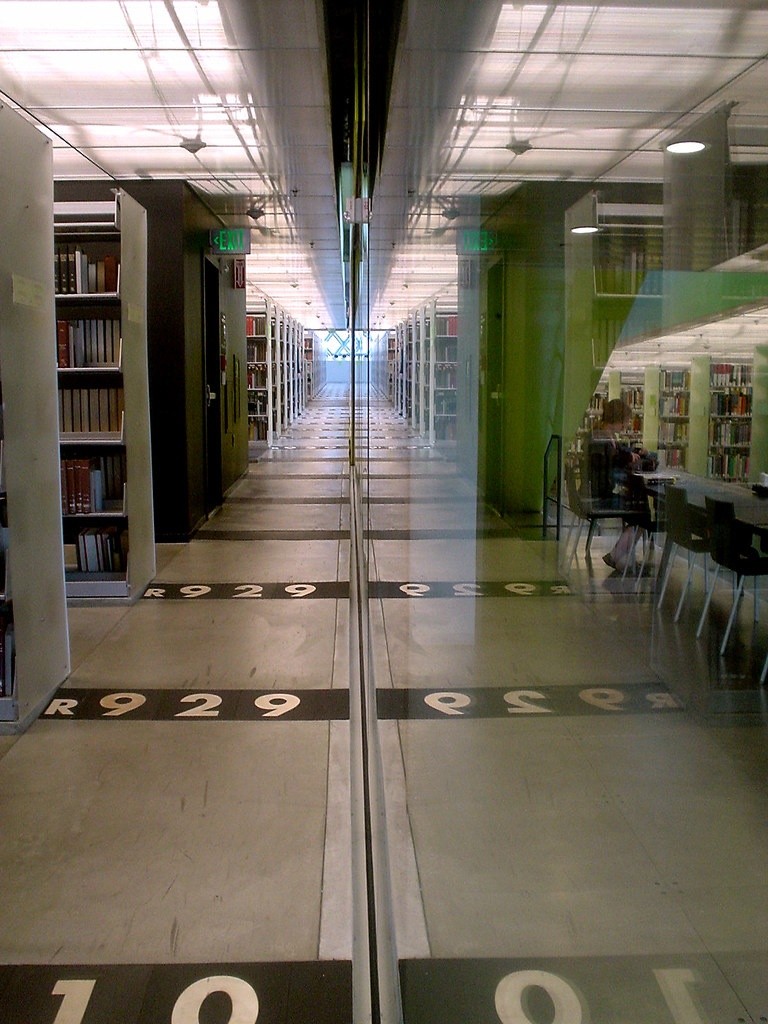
<box><xmin>543</xmin><ymin>434</ymin><xmax>768</xmax><ymax>684</ymax></box>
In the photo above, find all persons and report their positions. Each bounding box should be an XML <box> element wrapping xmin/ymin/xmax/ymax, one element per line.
<box><xmin>579</xmin><ymin>398</ymin><xmax>651</xmax><ymax>577</ymax></box>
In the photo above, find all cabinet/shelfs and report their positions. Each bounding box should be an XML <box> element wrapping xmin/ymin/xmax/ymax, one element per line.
<box><xmin>247</xmin><ymin>278</ymin><xmax>325</xmax><ymax>447</ymax></box>
<box><xmin>562</xmin><ymin>343</ymin><xmax>768</xmax><ymax>496</ymax></box>
<box><xmin>0</xmin><ymin>100</ymin><xmax>158</xmax><ymax>735</ymax></box>
<box><xmin>379</xmin><ymin>293</ymin><xmax>457</xmax><ymax>447</ymax></box>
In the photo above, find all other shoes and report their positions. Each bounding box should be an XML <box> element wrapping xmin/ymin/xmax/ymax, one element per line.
<box><xmin>602</xmin><ymin>553</ymin><xmax>616</xmax><ymax>569</ymax></box>
<box><xmin>615</xmin><ymin>560</ymin><xmax>651</xmax><ymax>578</ymax></box>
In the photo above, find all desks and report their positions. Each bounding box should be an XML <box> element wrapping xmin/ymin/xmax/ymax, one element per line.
<box><xmin>639</xmin><ymin>464</ymin><xmax>768</xmax><ymax>623</ymax></box>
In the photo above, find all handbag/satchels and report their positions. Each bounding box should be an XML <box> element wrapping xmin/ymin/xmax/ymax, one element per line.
<box><xmin>628</xmin><ymin>450</ymin><xmax>659</xmax><ymax>473</ymax></box>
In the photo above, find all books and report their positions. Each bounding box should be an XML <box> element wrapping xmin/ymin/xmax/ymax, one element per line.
<box><xmin>389</xmin><ymin>312</ymin><xmax>457</xmax><ymax>441</ymax></box>
<box><xmin>596</xmin><ymin>203</ymin><xmax>768</xmax><ymax>367</ymax></box>
<box><xmin>1</xmin><ymin>442</ymin><xmax>15</xmax><ymax>698</ymax></box>
<box><xmin>246</xmin><ymin>312</ymin><xmax>313</xmax><ymax>441</ymax></box>
<box><xmin>55</xmin><ymin>240</ymin><xmax>128</xmax><ymax>574</ymax></box>
<box><xmin>571</xmin><ymin>363</ymin><xmax>753</xmax><ymax>493</ymax></box>
